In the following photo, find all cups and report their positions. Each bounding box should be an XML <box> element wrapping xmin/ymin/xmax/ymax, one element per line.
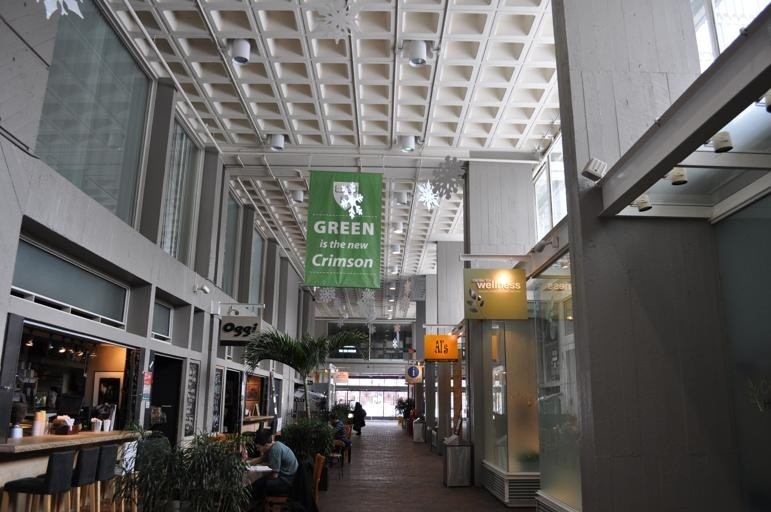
<box><xmin>103</xmin><ymin>419</ymin><xmax>110</xmax><ymax>432</ymax></box>
<box><xmin>91</xmin><ymin>419</ymin><xmax>102</xmax><ymax>432</ymax></box>
<box><xmin>32</xmin><ymin>410</ymin><xmax>48</xmax><ymax>436</ymax></box>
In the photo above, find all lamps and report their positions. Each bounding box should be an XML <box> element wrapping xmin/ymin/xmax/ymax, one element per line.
<box><xmin>628</xmin><ymin>82</ymin><xmax>771</xmax><ymax>218</ymax></box>
<box><xmin>20</xmin><ymin>325</ymin><xmax>99</xmax><ymax>361</ymax></box>
<box><xmin>192</xmin><ymin>283</ymin><xmax>210</xmax><ymax>296</ymax></box>
<box><xmin>226</xmin><ymin>33</ymin><xmax>430</xmax><ymax>204</ymax></box>
<box><xmin>385</xmin><ymin>190</ymin><xmax>409</xmax><ymax>322</ymax></box>
<box><xmin>582</xmin><ymin>157</ymin><xmax>609</xmax><ymax>183</ymax></box>
<box><xmin>534</xmin><ymin>235</ymin><xmax>561</xmax><ymax>254</ymax></box>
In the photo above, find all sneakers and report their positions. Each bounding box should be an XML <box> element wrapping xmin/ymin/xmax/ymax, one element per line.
<box><xmin>356</xmin><ymin>432</ymin><xmax>361</xmax><ymax>435</ymax></box>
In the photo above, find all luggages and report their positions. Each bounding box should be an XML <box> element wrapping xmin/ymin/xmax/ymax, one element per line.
<box><xmin>317</xmin><ymin>466</ymin><xmax>328</xmax><ymax>490</ymax></box>
<box><xmin>341</xmin><ymin>445</ymin><xmax>351</xmax><ymax>464</ymax></box>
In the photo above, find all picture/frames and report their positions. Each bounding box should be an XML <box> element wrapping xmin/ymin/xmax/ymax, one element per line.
<box><xmin>91</xmin><ymin>369</ymin><xmax>125</xmax><ymax>412</ymax></box>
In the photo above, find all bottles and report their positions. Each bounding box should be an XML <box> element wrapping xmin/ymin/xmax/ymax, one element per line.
<box><xmin>10</xmin><ymin>422</ymin><xmax>23</xmax><ymax>438</ymax></box>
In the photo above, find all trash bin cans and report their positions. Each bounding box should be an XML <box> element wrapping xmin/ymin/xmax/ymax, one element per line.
<box><xmin>413</xmin><ymin>417</ymin><xmax>426</xmax><ymax>443</ymax></box>
<box><xmin>440</xmin><ymin>434</ymin><xmax>473</xmax><ymax>488</ymax></box>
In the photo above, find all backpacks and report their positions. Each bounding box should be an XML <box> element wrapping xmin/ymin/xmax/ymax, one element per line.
<box><xmin>357</xmin><ymin>409</ymin><xmax>366</xmax><ymax>420</ymax></box>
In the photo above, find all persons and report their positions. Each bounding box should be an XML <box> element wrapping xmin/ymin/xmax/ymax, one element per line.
<box><xmin>329</xmin><ymin>413</ymin><xmax>347</xmax><ymax>443</ymax></box>
<box><xmin>250</xmin><ymin>430</ymin><xmax>302</xmax><ymax>511</ymax></box>
<box><xmin>137</xmin><ymin>422</ymin><xmax>172</xmax><ymax>478</ymax></box>
<box><xmin>351</xmin><ymin>401</ymin><xmax>366</xmax><ymax>435</ymax></box>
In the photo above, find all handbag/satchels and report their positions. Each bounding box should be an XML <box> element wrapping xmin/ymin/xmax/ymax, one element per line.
<box><xmin>360</xmin><ymin>421</ymin><xmax>365</xmax><ymax>427</ymax></box>
<box><xmin>353</xmin><ymin>422</ymin><xmax>361</xmax><ymax>431</ymax></box>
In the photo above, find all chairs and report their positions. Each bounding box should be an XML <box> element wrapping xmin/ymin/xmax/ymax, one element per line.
<box><xmin>0</xmin><ymin>417</ymin><xmax>357</xmax><ymax>512</ymax></box>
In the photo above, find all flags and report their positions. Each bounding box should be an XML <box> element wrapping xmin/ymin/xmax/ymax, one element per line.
<box><xmin>304</xmin><ymin>170</ymin><xmax>385</xmax><ymax>289</ymax></box>
